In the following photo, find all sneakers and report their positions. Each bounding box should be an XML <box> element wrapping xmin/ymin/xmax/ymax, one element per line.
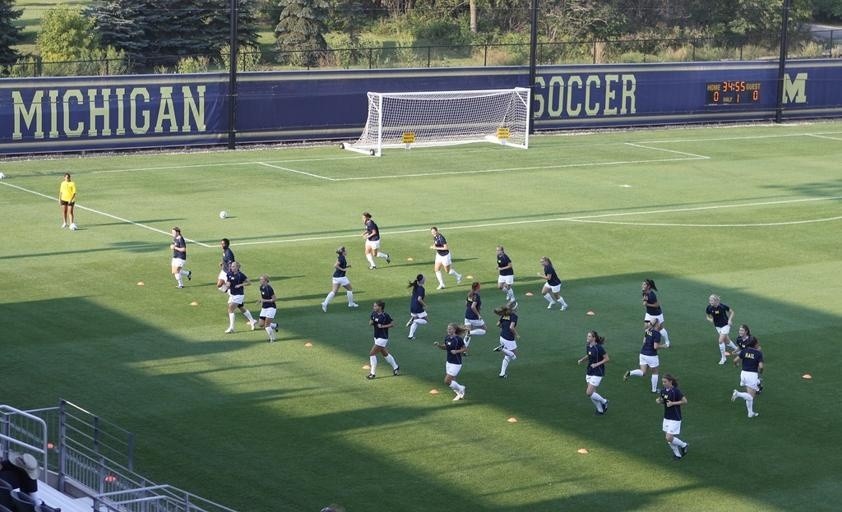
<box><xmin>651</xmin><ymin>389</ymin><xmax>661</xmax><ymax>394</ymax></box>
<box><xmin>463</xmin><ymin>352</ymin><xmax>473</xmax><ymax>357</ymax></box>
<box><xmin>457</xmin><ymin>274</ymin><xmax>462</xmax><ymax>284</ymax></box>
<box><xmin>436</xmin><ymin>285</ymin><xmax>445</xmax><ymax>289</ymax></box>
<box><xmin>718</xmin><ymin>358</ymin><xmax>727</xmax><ymax>364</ymax></box>
<box><xmin>560</xmin><ymin>305</ymin><xmax>568</xmax><ymax>311</ymax></box>
<box><xmin>321</xmin><ymin>302</ymin><xmax>327</xmax><ymax>312</ymax></box>
<box><xmin>348</xmin><ymin>303</ymin><xmax>359</xmax><ymax>307</ymax></box>
<box><xmin>671</xmin><ymin>455</ymin><xmax>682</xmax><ymax>462</ymax></box>
<box><xmin>592</xmin><ymin>409</ymin><xmax>604</xmax><ymax>416</ymax></box>
<box><xmin>665</xmin><ymin>341</ymin><xmax>670</xmax><ymax>348</ymax></box>
<box><xmin>465</xmin><ymin>331</ymin><xmax>470</xmax><ymax>342</ymax></box>
<box><xmin>681</xmin><ymin>443</ymin><xmax>688</xmax><ymax>454</ymax></box>
<box><xmin>506</xmin><ymin>293</ymin><xmax>510</xmax><ymax>301</ymax></box>
<box><xmin>408</xmin><ymin>336</ymin><xmax>416</xmax><ymax>341</ymax></box>
<box><xmin>275</xmin><ymin>322</ymin><xmax>278</xmax><ymax>333</ymax></box>
<box><xmin>365</xmin><ymin>374</ymin><xmax>376</xmax><ymax>380</ymax></box>
<box><xmin>224</xmin><ymin>328</ymin><xmax>234</xmax><ymax>333</ymax></box>
<box><xmin>267</xmin><ymin>339</ymin><xmax>276</xmax><ymax>343</ymax></box>
<box><xmin>493</xmin><ymin>345</ymin><xmax>504</xmax><ymax>352</ymax></box>
<box><xmin>386</xmin><ymin>254</ymin><xmax>391</xmax><ymax>264</ymax></box>
<box><xmin>367</xmin><ymin>265</ymin><xmax>376</xmax><ymax>270</ymax></box>
<box><xmin>406</xmin><ymin>317</ymin><xmax>415</xmax><ymax>327</ymax></box>
<box><xmin>393</xmin><ymin>366</ymin><xmax>399</xmax><ymax>376</ymax></box>
<box><xmin>602</xmin><ymin>399</ymin><xmax>609</xmax><ymax>414</ymax></box>
<box><xmin>459</xmin><ymin>385</ymin><xmax>466</xmax><ymax>398</ymax></box>
<box><xmin>748</xmin><ymin>412</ymin><xmax>759</xmax><ymax>418</ymax></box>
<box><xmin>250</xmin><ymin>320</ymin><xmax>258</xmax><ymax>331</ymax></box>
<box><xmin>453</xmin><ymin>394</ymin><xmax>460</xmax><ymax>402</ymax></box>
<box><xmin>498</xmin><ymin>373</ymin><xmax>508</xmax><ymax>379</ymax></box>
<box><xmin>623</xmin><ymin>370</ymin><xmax>630</xmax><ymax>383</ymax></box>
<box><xmin>175</xmin><ymin>285</ymin><xmax>184</xmax><ymax>289</ymax></box>
<box><xmin>547</xmin><ymin>301</ymin><xmax>556</xmax><ymax>310</ymax></box>
<box><xmin>187</xmin><ymin>271</ymin><xmax>192</xmax><ymax>281</ymax></box>
<box><xmin>61</xmin><ymin>223</ymin><xmax>67</xmax><ymax>229</ymax></box>
<box><xmin>731</xmin><ymin>389</ymin><xmax>737</xmax><ymax>402</ymax></box>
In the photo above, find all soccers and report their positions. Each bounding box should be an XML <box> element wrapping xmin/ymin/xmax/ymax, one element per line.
<box><xmin>220</xmin><ymin>211</ymin><xmax>229</xmax><ymax>220</ymax></box>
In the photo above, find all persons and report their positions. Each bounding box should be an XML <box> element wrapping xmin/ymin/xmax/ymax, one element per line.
<box><xmin>360</xmin><ymin>212</ymin><xmax>391</xmax><ymax>270</ymax></box>
<box><xmin>577</xmin><ymin>330</ymin><xmax>610</xmax><ymax>417</ymax></box>
<box><xmin>462</xmin><ymin>282</ymin><xmax>488</xmax><ymax>357</ymax></box>
<box><xmin>224</xmin><ymin>262</ymin><xmax>258</xmax><ymax>334</ymax></box>
<box><xmin>731</xmin><ymin>335</ymin><xmax>764</xmax><ymax>418</ymax></box>
<box><xmin>430</xmin><ymin>227</ymin><xmax>463</xmax><ymax>290</ymax></box>
<box><xmin>434</xmin><ymin>322</ymin><xmax>468</xmax><ymax>401</ymax></box>
<box><xmin>641</xmin><ymin>278</ymin><xmax>671</xmax><ymax>348</ymax></box>
<box><xmin>169</xmin><ymin>227</ymin><xmax>192</xmax><ymax>289</ymax></box>
<box><xmin>0</xmin><ymin>451</ymin><xmax>41</xmax><ymax>501</ymax></box>
<box><xmin>622</xmin><ymin>318</ymin><xmax>661</xmax><ymax>393</ymax></box>
<box><xmin>257</xmin><ymin>274</ymin><xmax>279</xmax><ymax>343</ymax></box>
<box><xmin>58</xmin><ymin>173</ymin><xmax>78</xmax><ymax>230</ymax></box>
<box><xmin>405</xmin><ymin>274</ymin><xmax>429</xmax><ymax>340</ymax></box>
<box><xmin>496</xmin><ymin>245</ymin><xmax>516</xmax><ymax>300</ymax></box>
<box><xmin>493</xmin><ymin>301</ymin><xmax>520</xmax><ymax>379</ymax></box>
<box><xmin>537</xmin><ymin>256</ymin><xmax>569</xmax><ymax>311</ymax></box>
<box><xmin>365</xmin><ymin>300</ymin><xmax>401</xmax><ymax>380</ymax></box>
<box><xmin>731</xmin><ymin>324</ymin><xmax>765</xmax><ymax>394</ymax></box>
<box><xmin>656</xmin><ymin>373</ymin><xmax>690</xmax><ymax>462</ymax></box>
<box><xmin>705</xmin><ymin>294</ymin><xmax>738</xmax><ymax>365</ymax></box>
<box><xmin>321</xmin><ymin>247</ymin><xmax>360</xmax><ymax>313</ymax></box>
<box><xmin>217</xmin><ymin>239</ymin><xmax>235</xmax><ymax>293</ymax></box>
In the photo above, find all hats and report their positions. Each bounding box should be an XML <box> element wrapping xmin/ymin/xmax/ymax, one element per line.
<box><xmin>8</xmin><ymin>448</ymin><xmax>41</xmax><ymax>480</ymax></box>
